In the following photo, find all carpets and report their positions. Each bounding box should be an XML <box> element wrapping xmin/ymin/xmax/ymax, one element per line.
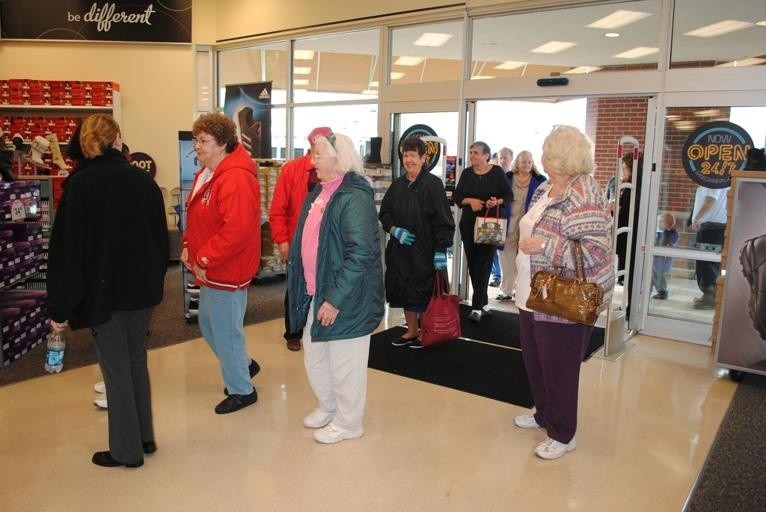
<box><xmin>0</xmin><ymin>265</ymin><xmax>307</xmax><ymax>389</ymax></box>
<box><xmin>455</xmin><ymin>301</ymin><xmax>607</xmax><ymax>359</ymax></box>
<box><xmin>684</xmin><ymin>376</ymin><xmax>766</xmax><ymax>512</ymax></box>
<box><xmin>348</xmin><ymin>324</ymin><xmax>539</xmax><ymax>410</ymax></box>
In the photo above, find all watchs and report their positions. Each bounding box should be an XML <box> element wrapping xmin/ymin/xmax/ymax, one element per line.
<box><xmin>541</xmin><ymin>239</ymin><xmax>548</xmax><ymax>254</ymax></box>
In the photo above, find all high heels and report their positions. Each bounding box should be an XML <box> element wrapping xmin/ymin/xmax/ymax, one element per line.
<box><xmin>1</xmin><ymin>127</ymin><xmax>73</xmax><ymax>182</ymax></box>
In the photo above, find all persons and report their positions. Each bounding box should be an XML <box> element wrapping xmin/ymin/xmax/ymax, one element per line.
<box><xmin>605</xmin><ymin>149</ymin><xmax>679</xmax><ymax>320</ymax></box>
<box><xmin>287</xmin><ymin>132</ymin><xmax>382</xmax><ymax>445</ymax></box>
<box><xmin>379</xmin><ymin>137</ymin><xmax>455</xmax><ymax>348</ymax></box>
<box><xmin>511</xmin><ymin>125</ymin><xmax>615</xmax><ymax>461</ymax></box>
<box><xmin>269</xmin><ymin>126</ymin><xmax>332</xmax><ymax>351</ymax></box>
<box><xmin>64</xmin><ymin>123</ymin><xmax>108</xmax><ymax>413</ymax></box>
<box><xmin>452</xmin><ymin>141</ymin><xmax>548</xmax><ymax>323</ymax></box>
<box><xmin>690</xmin><ymin>183</ymin><xmax>730</xmax><ymax>309</ymax></box>
<box><xmin>180</xmin><ymin>113</ymin><xmax>260</xmax><ymax>415</ymax></box>
<box><xmin>45</xmin><ymin>113</ymin><xmax>169</xmax><ymax>468</ymax></box>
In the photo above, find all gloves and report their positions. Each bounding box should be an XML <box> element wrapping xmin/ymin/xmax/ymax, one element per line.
<box><xmin>389</xmin><ymin>227</ymin><xmax>415</xmax><ymax>246</ymax></box>
<box><xmin>433</xmin><ymin>252</ymin><xmax>448</xmax><ymax>271</ymax></box>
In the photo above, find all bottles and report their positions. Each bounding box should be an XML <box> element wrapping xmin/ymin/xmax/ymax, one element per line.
<box><xmin>43</xmin><ymin>329</ymin><xmax>66</xmax><ymax>374</ymax></box>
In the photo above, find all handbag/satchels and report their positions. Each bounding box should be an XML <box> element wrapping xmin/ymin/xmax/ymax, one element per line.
<box><xmin>473</xmin><ymin>201</ymin><xmax>508</xmax><ymax>245</ymax></box>
<box><xmin>526</xmin><ymin>243</ymin><xmax>603</xmax><ymax>327</ymax></box>
<box><xmin>422</xmin><ymin>271</ymin><xmax>462</xmax><ymax>347</ymax></box>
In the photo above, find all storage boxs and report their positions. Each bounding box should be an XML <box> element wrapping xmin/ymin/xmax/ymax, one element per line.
<box><xmin>361</xmin><ymin>161</ymin><xmax>394</xmax><ymax>221</ymax></box>
<box><xmin>0</xmin><ymin>78</ymin><xmax>125</xmax><ymax>370</ymax></box>
<box><xmin>243</xmin><ymin>158</ymin><xmax>294</xmax><ymax>281</ymax></box>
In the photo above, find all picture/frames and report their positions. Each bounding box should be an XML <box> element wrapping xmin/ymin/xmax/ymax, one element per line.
<box><xmin>713</xmin><ymin>177</ymin><xmax>766</xmax><ymax>376</ymax></box>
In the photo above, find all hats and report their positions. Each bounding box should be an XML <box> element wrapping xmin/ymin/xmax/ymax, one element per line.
<box><xmin>308</xmin><ymin>127</ymin><xmax>332</xmax><ymax>145</ymax></box>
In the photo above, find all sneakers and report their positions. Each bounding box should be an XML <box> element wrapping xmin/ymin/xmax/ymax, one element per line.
<box><xmin>489</xmin><ymin>278</ymin><xmax>500</xmax><ymax>287</ymax></box>
<box><xmin>215</xmin><ymin>385</ymin><xmax>257</xmax><ymax>413</ymax></box>
<box><xmin>739</xmin><ymin>234</ymin><xmax>766</xmax><ymax>342</ymax></box>
<box><xmin>695</xmin><ymin>297</ymin><xmax>716</xmax><ymax>310</ymax></box>
<box><xmin>92</xmin><ymin>452</ymin><xmax>143</xmax><ymax>468</ymax></box>
<box><xmin>535</xmin><ymin>437</ymin><xmax>575</xmax><ymax>460</ymax></box>
<box><xmin>468</xmin><ymin>306</ymin><xmax>492</xmax><ymax>324</ymax></box>
<box><xmin>188</xmin><ymin>281</ymin><xmax>199</xmax><ymax>311</ymax></box>
<box><xmin>303</xmin><ymin>408</ymin><xmax>334</xmax><ymax>428</ymax></box>
<box><xmin>95</xmin><ymin>380</ymin><xmax>107</xmax><ymax>394</ymax></box>
<box><xmin>514</xmin><ymin>415</ymin><xmax>540</xmax><ymax>429</ymax></box>
<box><xmin>223</xmin><ymin>359</ymin><xmax>260</xmax><ymax>395</ymax></box>
<box><xmin>496</xmin><ymin>294</ymin><xmax>512</xmax><ymax>300</ymax></box>
<box><xmin>234</xmin><ymin>106</ymin><xmax>261</xmax><ymax>158</ymax></box>
<box><xmin>314</xmin><ymin>422</ymin><xmax>363</xmax><ymax>443</ymax></box>
<box><xmin>392</xmin><ymin>336</ymin><xmax>424</xmax><ymax>349</ymax></box>
<box><xmin>653</xmin><ymin>293</ymin><xmax>666</xmax><ymax>299</ymax></box>
<box><xmin>287</xmin><ymin>338</ymin><xmax>301</xmax><ymax>350</ymax></box>
<box><xmin>94</xmin><ymin>396</ymin><xmax>107</xmax><ymax>410</ymax></box>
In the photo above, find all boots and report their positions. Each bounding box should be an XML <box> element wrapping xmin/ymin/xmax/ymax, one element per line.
<box><xmin>366</xmin><ymin>137</ymin><xmax>382</xmax><ymax>164</ymax></box>
